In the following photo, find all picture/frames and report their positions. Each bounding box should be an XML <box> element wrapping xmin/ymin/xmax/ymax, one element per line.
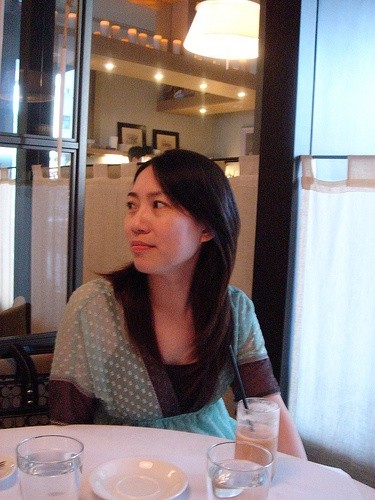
<box><xmin>117</xmin><ymin>121</ymin><xmax>147</xmax><ymax>152</ymax></box>
<box><xmin>153</xmin><ymin>128</ymin><xmax>179</xmax><ymax>151</ymax></box>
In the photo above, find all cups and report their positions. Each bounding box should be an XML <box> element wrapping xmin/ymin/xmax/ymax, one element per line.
<box><xmin>206</xmin><ymin>440</ymin><xmax>274</xmax><ymax>500</ymax></box>
<box><xmin>108</xmin><ymin>135</ymin><xmax>118</xmax><ymax>149</ymax></box>
<box><xmin>15</xmin><ymin>434</ymin><xmax>84</xmax><ymax>500</ymax></box>
<box><xmin>234</xmin><ymin>396</ymin><xmax>281</xmax><ymax>467</ymax></box>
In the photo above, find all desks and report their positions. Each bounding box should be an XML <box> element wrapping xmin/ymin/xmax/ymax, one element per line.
<box><xmin>0</xmin><ymin>425</ymin><xmax>375</xmax><ymax>500</ymax></box>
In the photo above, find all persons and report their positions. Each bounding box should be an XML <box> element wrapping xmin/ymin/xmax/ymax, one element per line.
<box><xmin>128</xmin><ymin>146</ymin><xmax>154</xmax><ymax>163</ymax></box>
<box><xmin>46</xmin><ymin>148</ymin><xmax>310</xmax><ymax>460</ymax></box>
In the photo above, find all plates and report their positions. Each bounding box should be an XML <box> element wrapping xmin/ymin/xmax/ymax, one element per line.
<box><xmin>0</xmin><ymin>453</ymin><xmax>17</xmax><ymax>480</ymax></box>
<box><xmin>90</xmin><ymin>457</ymin><xmax>190</xmax><ymax>500</ymax></box>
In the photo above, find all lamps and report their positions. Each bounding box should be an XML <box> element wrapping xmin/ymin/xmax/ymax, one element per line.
<box><xmin>183</xmin><ymin>0</ymin><xmax>264</xmax><ymax>62</ymax></box>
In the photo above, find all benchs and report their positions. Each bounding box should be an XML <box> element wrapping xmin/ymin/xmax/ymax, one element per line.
<box><xmin>0</xmin><ymin>330</ymin><xmax>57</xmax><ymax>429</ymax></box>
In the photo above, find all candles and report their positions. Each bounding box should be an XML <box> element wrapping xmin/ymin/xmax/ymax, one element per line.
<box><xmin>153</xmin><ymin>34</ymin><xmax>162</xmax><ymax>50</ymax></box>
<box><xmin>100</xmin><ymin>20</ymin><xmax>110</xmax><ymax>37</ymax></box>
<box><xmin>127</xmin><ymin>28</ymin><xmax>137</xmax><ymax>44</ymax></box>
<box><xmin>162</xmin><ymin>38</ymin><xmax>168</xmax><ymax>51</ymax></box>
<box><xmin>139</xmin><ymin>33</ymin><xmax>147</xmax><ymax>46</ymax></box>
<box><xmin>112</xmin><ymin>25</ymin><xmax>120</xmax><ymax>39</ymax></box>
